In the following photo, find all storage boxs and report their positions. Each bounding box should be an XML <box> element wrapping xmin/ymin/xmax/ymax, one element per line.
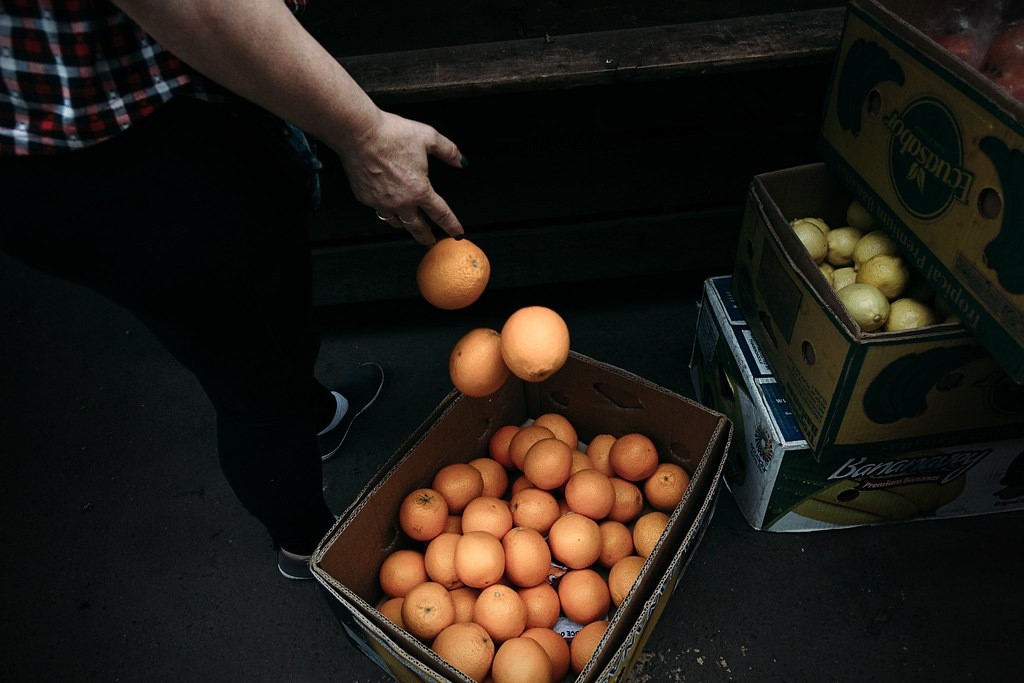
<box><xmin>818</xmin><ymin>0</ymin><xmax>1024</xmax><ymax>386</ymax></box>
<box><xmin>728</xmin><ymin>166</ymin><xmax>1024</xmax><ymax>462</ymax></box>
<box><xmin>688</xmin><ymin>276</ymin><xmax>1024</xmax><ymax>533</ymax></box>
<box><xmin>310</xmin><ymin>349</ymin><xmax>733</xmax><ymax>683</ymax></box>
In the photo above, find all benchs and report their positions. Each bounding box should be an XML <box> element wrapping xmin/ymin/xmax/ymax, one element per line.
<box><xmin>334</xmin><ymin>5</ymin><xmax>845</xmax><ymax>100</ymax></box>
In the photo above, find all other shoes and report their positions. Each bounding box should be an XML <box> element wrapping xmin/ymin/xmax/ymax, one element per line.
<box><xmin>278</xmin><ymin>514</ymin><xmax>342</xmax><ymax>581</ymax></box>
<box><xmin>317</xmin><ymin>363</ymin><xmax>385</xmax><ymax>459</ymax></box>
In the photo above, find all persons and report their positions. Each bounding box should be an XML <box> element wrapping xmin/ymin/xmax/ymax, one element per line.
<box><xmin>0</xmin><ymin>0</ymin><xmax>465</xmax><ymax>580</ymax></box>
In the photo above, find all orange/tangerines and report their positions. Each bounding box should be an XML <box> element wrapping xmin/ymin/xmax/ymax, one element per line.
<box><xmin>500</xmin><ymin>307</ymin><xmax>570</xmax><ymax>381</ymax></box>
<box><xmin>449</xmin><ymin>328</ymin><xmax>509</xmax><ymax>397</ymax></box>
<box><xmin>417</xmin><ymin>237</ymin><xmax>489</xmax><ymax>310</ymax></box>
<box><xmin>376</xmin><ymin>413</ymin><xmax>689</xmax><ymax>683</ymax></box>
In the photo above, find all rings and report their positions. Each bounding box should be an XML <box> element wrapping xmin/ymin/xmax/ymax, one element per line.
<box><xmin>376</xmin><ymin>211</ymin><xmax>396</xmax><ymax>222</ymax></box>
<box><xmin>399</xmin><ymin>216</ymin><xmax>418</xmax><ymax>225</ymax></box>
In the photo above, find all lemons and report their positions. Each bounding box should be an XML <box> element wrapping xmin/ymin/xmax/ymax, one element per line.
<box><xmin>787</xmin><ymin>198</ymin><xmax>963</xmax><ymax>332</ymax></box>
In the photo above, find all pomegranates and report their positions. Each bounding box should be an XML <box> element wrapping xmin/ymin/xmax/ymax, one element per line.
<box><xmin>921</xmin><ymin>17</ymin><xmax>1024</xmax><ymax>109</ymax></box>
